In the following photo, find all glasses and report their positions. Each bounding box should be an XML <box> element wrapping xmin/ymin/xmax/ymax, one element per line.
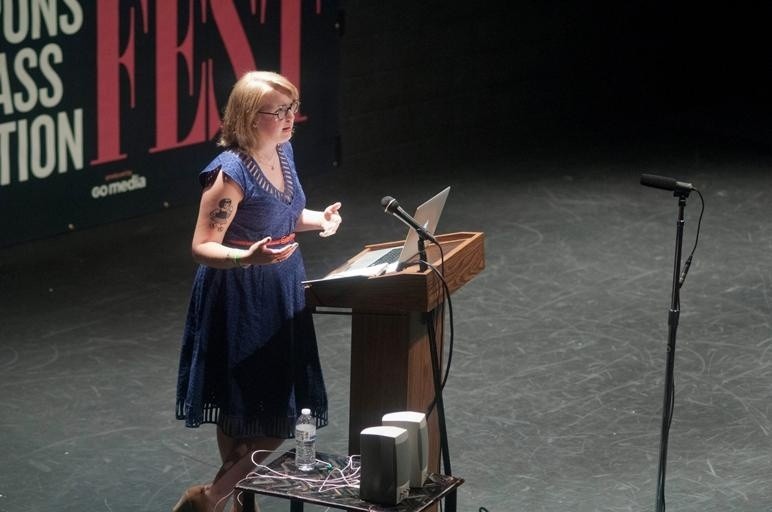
<box><xmin>258</xmin><ymin>100</ymin><xmax>299</xmax><ymax>120</ymax></box>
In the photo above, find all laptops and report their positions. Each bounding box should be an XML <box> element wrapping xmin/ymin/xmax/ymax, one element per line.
<box><xmin>347</xmin><ymin>186</ymin><xmax>452</xmax><ymax>274</ymax></box>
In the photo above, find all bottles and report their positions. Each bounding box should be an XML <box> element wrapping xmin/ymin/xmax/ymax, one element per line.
<box><xmin>294</xmin><ymin>408</ymin><xmax>317</xmax><ymax>472</ymax></box>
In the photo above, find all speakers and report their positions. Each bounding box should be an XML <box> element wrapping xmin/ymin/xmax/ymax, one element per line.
<box><xmin>381</xmin><ymin>411</ymin><xmax>428</xmax><ymax>489</ymax></box>
<box><xmin>360</xmin><ymin>427</ymin><xmax>411</xmax><ymax>505</ymax></box>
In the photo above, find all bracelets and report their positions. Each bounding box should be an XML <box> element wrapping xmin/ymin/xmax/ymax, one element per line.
<box><xmin>235</xmin><ymin>254</ymin><xmax>251</xmax><ymax>270</ymax></box>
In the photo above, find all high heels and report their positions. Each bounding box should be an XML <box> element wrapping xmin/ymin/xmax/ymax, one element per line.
<box><xmin>171</xmin><ymin>485</ymin><xmax>208</xmax><ymax>512</ymax></box>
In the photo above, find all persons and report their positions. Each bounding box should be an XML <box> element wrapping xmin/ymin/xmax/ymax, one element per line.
<box><xmin>173</xmin><ymin>71</ymin><xmax>342</xmax><ymax>512</ymax></box>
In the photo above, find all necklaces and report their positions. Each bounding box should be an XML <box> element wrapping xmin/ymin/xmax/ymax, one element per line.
<box><xmin>257</xmin><ymin>153</ymin><xmax>277</xmax><ymax>170</ymax></box>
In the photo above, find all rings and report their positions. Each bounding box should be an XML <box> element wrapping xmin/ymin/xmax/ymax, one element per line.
<box><xmin>278</xmin><ymin>259</ymin><xmax>281</xmax><ymax>263</ymax></box>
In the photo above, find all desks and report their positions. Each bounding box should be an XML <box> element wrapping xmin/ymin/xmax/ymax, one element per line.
<box><xmin>235</xmin><ymin>448</ymin><xmax>464</xmax><ymax>511</ymax></box>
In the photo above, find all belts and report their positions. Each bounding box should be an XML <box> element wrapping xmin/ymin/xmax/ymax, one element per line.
<box><xmin>223</xmin><ymin>233</ymin><xmax>296</xmax><ymax>246</ymax></box>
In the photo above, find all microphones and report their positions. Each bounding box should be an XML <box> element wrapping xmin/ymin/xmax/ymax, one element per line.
<box><xmin>381</xmin><ymin>196</ymin><xmax>437</xmax><ymax>244</ymax></box>
<box><xmin>637</xmin><ymin>172</ymin><xmax>694</xmax><ymax>191</ymax></box>
